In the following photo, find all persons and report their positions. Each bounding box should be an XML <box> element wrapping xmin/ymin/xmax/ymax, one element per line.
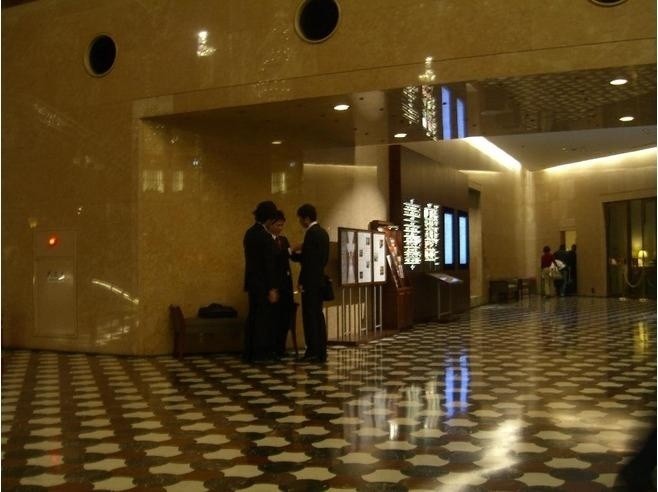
<box><xmin>239</xmin><ymin>199</ymin><xmax>284</xmax><ymax>365</ymax></box>
<box><xmin>552</xmin><ymin>244</ymin><xmax>570</xmax><ymax>297</ymax></box>
<box><xmin>260</xmin><ymin>209</ymin><xmax>296</xmax><ymax>360</ymax></box>
<box><xmin>569</xmin><ymin>243</ymin><xmax>577</xmax><ymax>294</ymax></box>
<box><xmin>287</xmin><ymin>203</ymin><xmax>330</xmax><ymax>362</ymax></box>
<box><xmin>540</xmin><ymin>245</ymin><xmax>559</xmax><ymax>296</ymax></box>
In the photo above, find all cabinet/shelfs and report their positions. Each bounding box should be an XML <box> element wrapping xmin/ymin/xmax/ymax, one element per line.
<box><xmin>384</xmin><ymin>288</ymin><xmax>414</xmax><ymax>329</ymax></box>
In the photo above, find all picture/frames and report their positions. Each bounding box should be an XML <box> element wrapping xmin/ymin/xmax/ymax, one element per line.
<box><xmin>338</xmin><ymin>227</ymin><xmax>388</xmax><ymax>286</ymax></box>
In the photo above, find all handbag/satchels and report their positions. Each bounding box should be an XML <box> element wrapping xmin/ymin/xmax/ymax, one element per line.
<box><xmin>324</xmin><ymin>276</ymin><xmax>334</xmax><ymax>301</ymax></box>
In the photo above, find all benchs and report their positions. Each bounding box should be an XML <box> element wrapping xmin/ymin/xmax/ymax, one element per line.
<box><xmin>490</xmin><ymin>276</ymin><xmax>532</xmax><ymax>300</ymax></box>
<box><xmin>167</xmin><ymin>304</ymin><xmax>249</xmax><ymax>359</ymax></box>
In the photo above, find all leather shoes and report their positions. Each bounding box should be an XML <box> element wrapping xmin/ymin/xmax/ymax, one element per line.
<box><xmin>300</xmin><ymin>354</ymin><xmax>324</xmax><ymax>361</ymax></box>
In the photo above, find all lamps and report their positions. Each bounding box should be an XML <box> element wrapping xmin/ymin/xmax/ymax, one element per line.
<box><xmin>638</xmin><ymin>248</ymin><xmax>648</xmax><ymax>264</ymax></box>
